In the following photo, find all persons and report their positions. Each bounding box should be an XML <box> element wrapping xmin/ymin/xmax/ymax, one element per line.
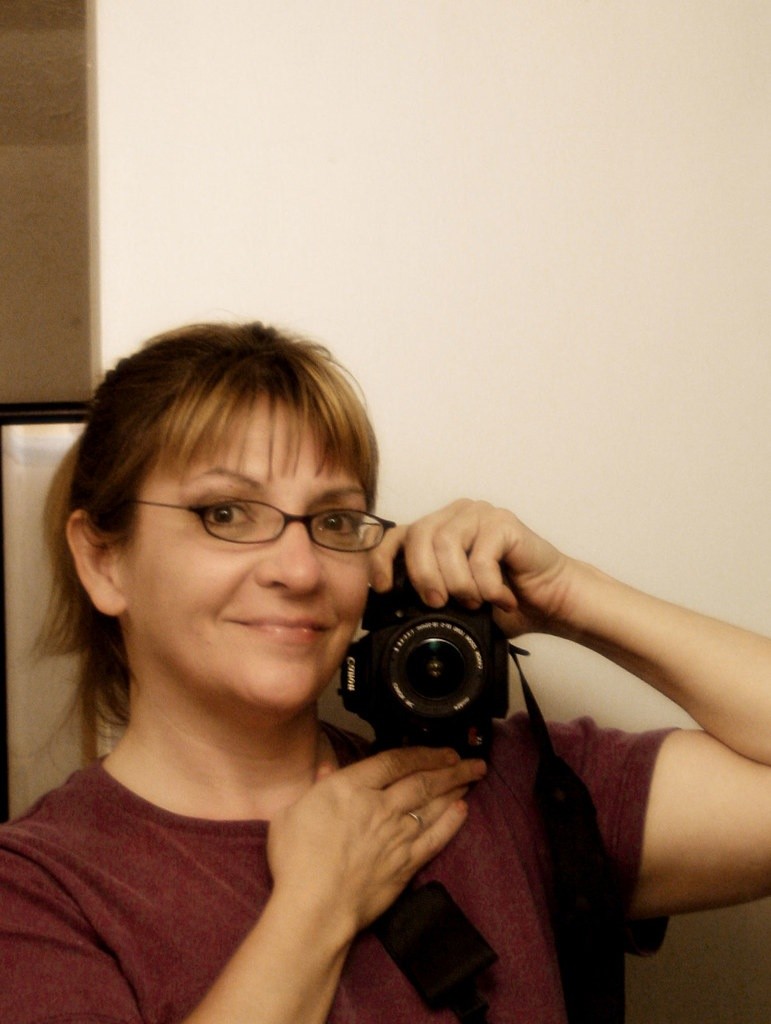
<box><xmin>0</xmin><ymin>318</ymin><xmax>770</xmax><ymax>1024</ymax></box>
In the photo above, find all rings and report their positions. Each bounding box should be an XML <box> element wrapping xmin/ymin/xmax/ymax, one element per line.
<box><xmin>407</xmin><ymin>811</ymin><xmax>423</xmax><ymax>837</ymax></box>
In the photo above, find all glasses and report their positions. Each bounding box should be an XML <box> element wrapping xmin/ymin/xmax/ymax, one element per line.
<box><xmin>134</xmin><ymin>498</ymin><xmax>396</xmax><ymax>553</ymax></box>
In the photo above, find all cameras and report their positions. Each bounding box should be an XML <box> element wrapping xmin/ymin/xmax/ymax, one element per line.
<box><xmin>342</xmin><ymin>547</ymin><xmax>511</xmax><ymax>786</ymax></box>
